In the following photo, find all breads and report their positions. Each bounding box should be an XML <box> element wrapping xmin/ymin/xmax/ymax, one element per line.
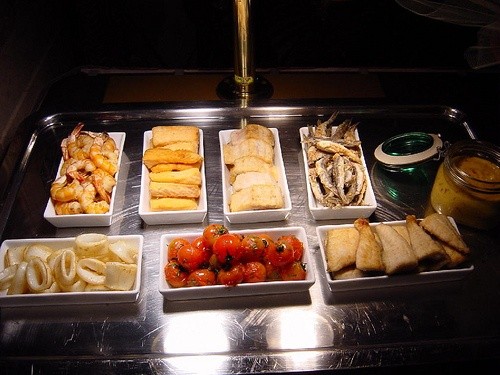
<box><xmin>143</xmin><ymin>126</ymin><xmax>204</xmax><ymax>210</ymax></box>
<box><xmin>223</xmin><ymin>124</ymin><xmax>282</xmax><ymax>212</ymax></box>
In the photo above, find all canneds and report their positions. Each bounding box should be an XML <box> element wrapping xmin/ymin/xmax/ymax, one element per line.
<box><xmin>373</xmin><ymin>132</ymin><xmax>500</xmax><ymax>230</ymax></box>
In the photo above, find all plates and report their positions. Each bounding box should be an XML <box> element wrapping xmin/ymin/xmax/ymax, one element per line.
<box><xmin>219</xmin><ymin>127</ymin><xmax>293</xmax><ymax>223</ymax></box>
<box><xmin>316</xmin><ymin>215</ymin><xmax>474</xmax><ymax>292</ymax></box>
<box><xmin>299</xmin><ymin>123</ymin><xmax>377</xmax><ymax>219</ymax></box>
<box><xmin>0</xmin><ymin>235</ymin><xmax>144</xmax><ymax>309</ymax></box>
<box><xmin>43</xmin><ymin>132</ymin><xmax>126</xmax><ymax>226</ymax></box>
<box><xmin>138</xmin><ymin>127</ymin><xmax>208</xmax><ymax>225</ymax></box>
<box><xmin>159</xmin><ymin>225</ymin><xmax>314</xmax><ymax>300</ymax></box>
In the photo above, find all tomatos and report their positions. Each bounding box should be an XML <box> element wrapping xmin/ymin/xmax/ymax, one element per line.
<box><xmin>164</xmin><ymin>224</ymin><xmax>307</xmax><ymax>288</ymax></box>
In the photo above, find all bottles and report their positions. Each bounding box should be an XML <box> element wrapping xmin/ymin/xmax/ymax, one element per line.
<box><xmin>430</xmin><ymin>139</ymin><xmax>500</xmax><ymax>227</ymax></box>
